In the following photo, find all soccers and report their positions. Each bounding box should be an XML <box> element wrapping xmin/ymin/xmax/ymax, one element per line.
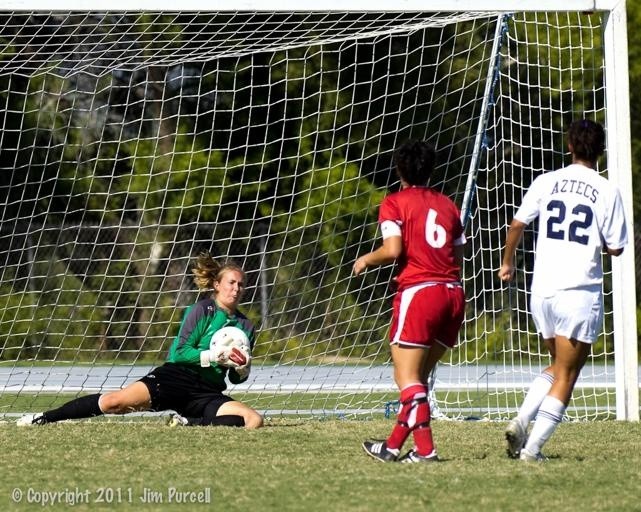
<box><xmin>210</xmin><ymin>326</ymin><xmax>250</xmax><ymax>367</ymax></box>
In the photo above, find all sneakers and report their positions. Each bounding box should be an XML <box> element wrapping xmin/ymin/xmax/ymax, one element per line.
<box><xmin>16</xmin><ymin>412</ymin><xmax>46</xmax><ymax>427</ymax></box>
<box><xmin>159</xmin><ymin>409</ymin><xmax>188</xmax><ymax>428</ymax></box>
<box><xmin>505</xmin><ymin>418</ymin><xmax>550</xmax><ymax>463</ymax></box>
<box><xmin>362</xmin><ymin>441</ymin><xmax>439</xmax><ymax>465</ymax></box>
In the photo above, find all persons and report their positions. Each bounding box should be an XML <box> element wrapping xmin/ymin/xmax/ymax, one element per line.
<box><xmin>497</xmin><ymin>117</ymin><xmax>629</xmax><ymax>463</ymax></box>
<box><xmin>353</xmin><ymin>137</ymin><xmax>467</xmax><ymax>465</ymax></box>
<box><xmin>15</xmin><ymin>251</ymin><xmax>264</xmax><ymax>428</ymax></box>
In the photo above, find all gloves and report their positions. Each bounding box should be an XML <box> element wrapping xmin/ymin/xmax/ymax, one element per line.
<box><xmin>200</xmin><ymin>333</ymin><xmax>252</xmax><ymax>380</ymax></box>
